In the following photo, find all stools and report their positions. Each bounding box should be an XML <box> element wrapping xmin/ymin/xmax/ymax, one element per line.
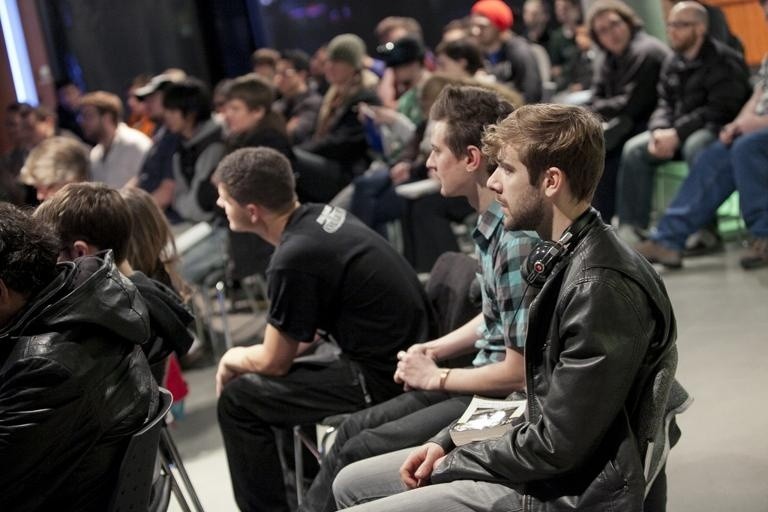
<box><xmin>651</xmin><ymin>156</ymin><xmax>748</xmax><ymax>252</ymax></box>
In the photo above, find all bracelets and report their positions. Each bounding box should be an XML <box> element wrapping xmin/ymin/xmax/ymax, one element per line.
<box><xmin>438</xmin><ymin>367</ymin><xmax>452</xmax><ymax>390</ymax></box>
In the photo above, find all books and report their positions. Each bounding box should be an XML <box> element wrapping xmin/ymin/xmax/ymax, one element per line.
<box><xmin>449</xmin><ymin>395</ymin><xmax>528</xmax><ymax>446</ymax></box>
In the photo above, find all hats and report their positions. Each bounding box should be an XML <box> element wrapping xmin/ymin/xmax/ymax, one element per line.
<box><xmin>135</xmin><ymin>74</ymin><xmax>174</xmax><ymax>99</ymax></box>
<box><xmin>472</xmin><ymin>1</ymin><xmax>514</xmax><ymax>32</ymax></box>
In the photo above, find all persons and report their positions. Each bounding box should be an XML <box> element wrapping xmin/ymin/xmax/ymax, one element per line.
<box><xmin>28</xmin><ymin>180</ymin><xmax>195</xmax><ymax>366</ymax></box>
<box><xmin>1</xmin><ymin>202</ymin><xmax>160</xmax><ymax>509</ymax></box>
<box><xmin>211</xmin><ymin>146</ymin><xmax>441</xmax><ymax>511</ymax></box>
<box><xmin>329</xmin><ymin>102</ymin><xmax>677</xmax><ymax>511</ymax></box>
<box><xmin>295</xmin><ymin>84</ymin><xmax>544</xmax><ymax>510</ymax></box>
<box><xmin>115</xmin><ymin>185</ymin><xmax>177</xmax><ymax>289</ymax></box>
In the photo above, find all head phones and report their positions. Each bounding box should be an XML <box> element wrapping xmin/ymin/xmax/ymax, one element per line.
<box><xmin>520</xmin><ymin>206</ymin><xmax>601</xmax><ymax>290</ymax></box>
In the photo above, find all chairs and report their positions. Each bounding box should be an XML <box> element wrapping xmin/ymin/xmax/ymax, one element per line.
<box><xmin>204</xmin><ymin>255</ymin><xmax>269</xmax><ymax>368</ymax></box>
<box><xmin>641</xmin><ymin>343</ymin><xmax>692</xmax><ymax>510</ymax></box>
<box><xmin>112</xmin><ymin>385</ymin><xmax>212</xmax><ymax>511</ymax></box>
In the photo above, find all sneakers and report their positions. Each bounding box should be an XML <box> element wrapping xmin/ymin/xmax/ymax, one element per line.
<box><xmin>633</xmin><ymin>232</ymin><xmax>764</xmax><ymax>268</ymax></box>
